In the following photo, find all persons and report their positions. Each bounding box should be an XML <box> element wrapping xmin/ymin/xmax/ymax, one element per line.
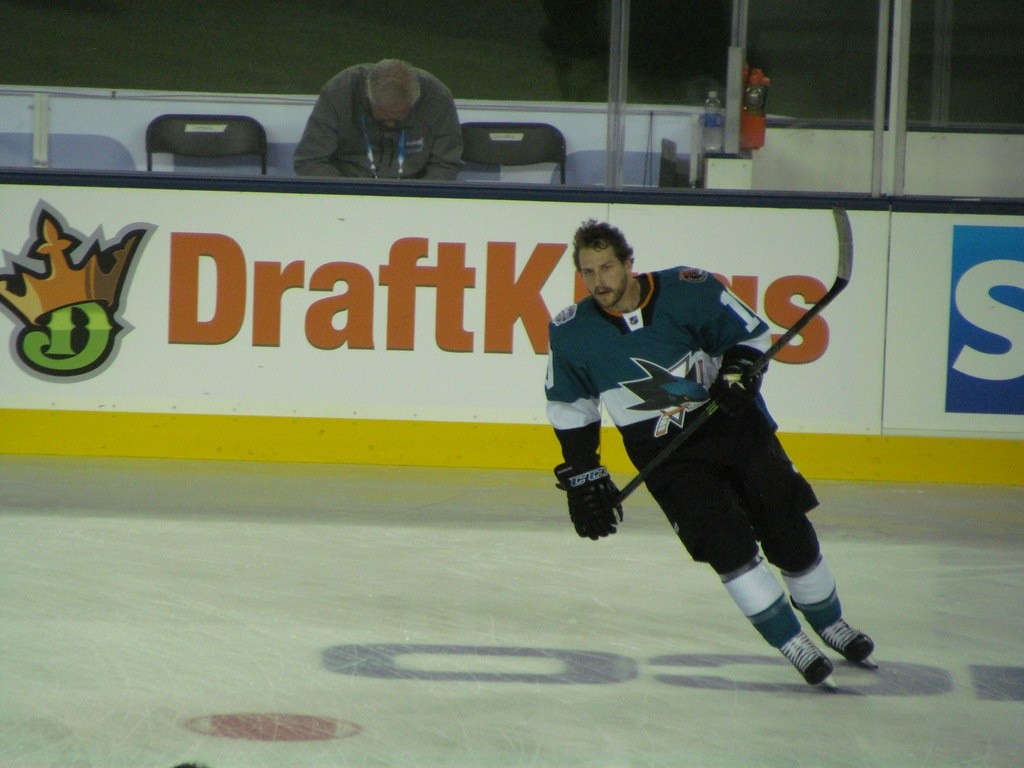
<box><xmin>544</xmin><ymin>220</ymin><xmax>879</xmax><ymax>692</ymax></box>
<box><xmin>293</xmin><ymin>57</ymin><xmax>466</xmax><ymax>182</ymax></box>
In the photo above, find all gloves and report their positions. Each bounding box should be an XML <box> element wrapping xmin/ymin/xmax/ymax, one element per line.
<box><xmin>710</xmin><ymin>367</ymin><xmax>754</xmax><ymax>419</ymax></box>
<box><xmin>555</xmin><ymin>454</ymin><xmax>623</xmax><ymax>540</ymax></box>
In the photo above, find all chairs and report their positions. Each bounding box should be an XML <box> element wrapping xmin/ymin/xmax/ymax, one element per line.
<box><xmin>458</xmin><ymin>122</ymin><xmax>567</xmax><ymax>186</ymax></box>
<box><xmin>145</xmin><ymin>113</ymin><xmax>266</xmax><ymax>176</ymax></box>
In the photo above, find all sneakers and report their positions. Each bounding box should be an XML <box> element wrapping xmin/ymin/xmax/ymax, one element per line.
<box><xmin>779</xmin><ymin>634</ymin><xmax>839</xmax><ymax>693</ymax></box>
<box><xmin>811</xmin><ymin>622</ymin><xmax>877</xmax><ymax>669</ymax></box>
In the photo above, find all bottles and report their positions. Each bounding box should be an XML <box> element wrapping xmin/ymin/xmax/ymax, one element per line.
<box><xmin>739</xmin><ymin>68</ymin><xmax>769</xmax><ymax>147</ymax></box>
<box><xmin>701</xmin><ymin>91</ymin><xmax>722</xmax><ymax>152</ymax></box>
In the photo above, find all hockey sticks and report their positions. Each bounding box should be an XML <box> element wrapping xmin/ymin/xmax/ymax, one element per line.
<box><xmin>611</xmin><ymin>204</ymin><xmax>854</xmax><ymax>511</ymax></box>
